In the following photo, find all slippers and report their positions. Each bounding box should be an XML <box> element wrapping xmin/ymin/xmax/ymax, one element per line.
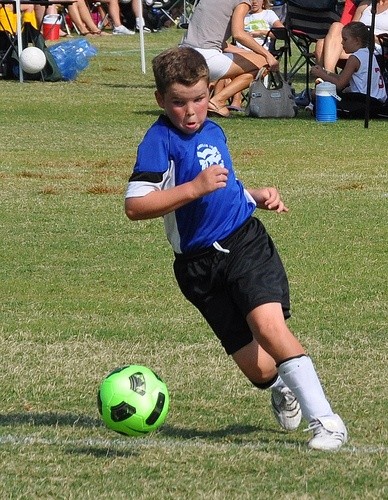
<box><xmin>59</xmin><ymin>33</ymin><xmax>74</xmax><ymax>38</ymax></box>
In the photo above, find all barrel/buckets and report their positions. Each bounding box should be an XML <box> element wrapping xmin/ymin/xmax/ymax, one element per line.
<box><xmin>315</xmin><ymin>81</ymin><xmax>341</xmax><ymax>122</ymax></box>
<box><xmin>42</xmin><ymin>14</ymin><xmax>62</xmax><ymax>40</ymax></box>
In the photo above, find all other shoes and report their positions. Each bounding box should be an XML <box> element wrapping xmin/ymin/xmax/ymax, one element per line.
<box><xmin>305</xmin><ymin>102</ymin><xmax>314</xmax><ymax>111</ymax></box>
<box><xmin>315</xmin><ymin>78</ymin><xmax>324</xmax><ymax>84</ymax></box>
<box><xmin>80</xmin><ymin>30</ymin><xmax>113</xmax><ymax>37</ymax></box>
<box><xmin>228</xmin><ymin>105</ymin><xmax>245</xmax><ymax>112</ymax></box>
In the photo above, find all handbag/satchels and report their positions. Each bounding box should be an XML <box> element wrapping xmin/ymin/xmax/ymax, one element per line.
<box><xmin>247</xmin><ymin>63</ymin><xmax>299</xmax><ymax>118</ymax></box>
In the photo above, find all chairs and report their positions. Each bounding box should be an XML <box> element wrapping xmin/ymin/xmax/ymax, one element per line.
<box><xmin>0</xmin><ymin>0</ymin><xmax>349</xmax><ymax>107</ymax></box>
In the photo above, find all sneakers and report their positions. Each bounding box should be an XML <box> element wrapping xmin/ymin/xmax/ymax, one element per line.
<box><xmin>113</xmin><ymin>25</ymin><xmax>135</xmax><ymax>36</ymax></box>
<box><xmin>135</xmin><ymin>24</ymin><xmax>158</xmax><ymax>33</ymax></box>
<box><xmin>302</xmin><ymin>414</ymin><xmax>347</xmax><ymax>452</ymax></box>
<box><xmin>271</xmin><ymin>386</ymin><xmax>302</xmax><ymax>431</ymax></box>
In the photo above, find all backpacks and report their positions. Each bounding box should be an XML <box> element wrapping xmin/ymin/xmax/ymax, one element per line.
<box><xmin>119</xmin><ymin>0</ymin><xmax>173</xmax><ymax>30</ymax></box>
<box><xmin>0</xmin><ymin>22</ymin><xmax>62</xmax><ymax>81</ymax></box>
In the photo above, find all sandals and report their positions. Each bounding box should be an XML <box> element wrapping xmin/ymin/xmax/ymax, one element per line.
<box><xmin>208</xmin><ymin>100</ymin><xmax>232</xmax><ymax>118</ymax></box>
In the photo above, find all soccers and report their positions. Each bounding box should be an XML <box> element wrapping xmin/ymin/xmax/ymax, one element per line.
<box><xmin>97</xmin><ymin>365</ymin><xmax>169</xmax><ymax>436</ymax></box>
<box><xmin>20</xmin><ymin>46</ymin><xmax>46</xmax><ymax>74</ymax></box>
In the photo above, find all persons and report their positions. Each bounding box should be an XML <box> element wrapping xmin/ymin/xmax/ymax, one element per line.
<box><xmin>226</xmin><ymin>0</ymin><xmax>285</xmax><ymax>111</ymax></box>
<box><xmin>0</xmin><ymin>0</ymin><xmax>151</xmax><ymax>38</ymax></box>
<box><xmin>183</xmin><ymin>0</ymin><xmax>279</xmax><ymax>117</ymax></box>
<box><xmin>124</xmin><ymin>47</ymin><xmax>348</xmax><ymax>451</ymax></box>
<box><xmin>310</xmin><ymin>0</ymin><xmax>388</xmax><ymax>98</ymax></box>
<box><xmin>311</xmin><ymin>21</ymin><xmax>387</xmax><ymax>102</ymax></box>
